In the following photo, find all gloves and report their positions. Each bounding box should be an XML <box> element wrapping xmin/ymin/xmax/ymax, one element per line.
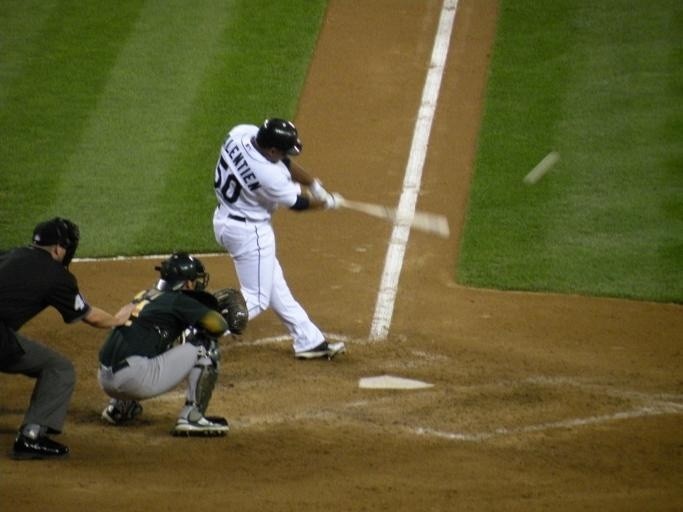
<box><xmin>309</xmin><ymin>178</ymin><xmax>343</xmax><ymax>209</ymax></box>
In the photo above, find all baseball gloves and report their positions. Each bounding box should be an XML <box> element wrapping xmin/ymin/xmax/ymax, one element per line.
<box><xmin>217</xmin><ymin>289</ymin><xmax>248</xmax><ymax>331</ymax></box>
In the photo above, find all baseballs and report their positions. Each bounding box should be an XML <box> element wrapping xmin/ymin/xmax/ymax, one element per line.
<box><xmin>523</xmin><ymin>149</ymin><xmax>559</xmax><ymax>187</ymax></box>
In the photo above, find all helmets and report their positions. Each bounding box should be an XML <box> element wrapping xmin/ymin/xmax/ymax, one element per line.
<box><xmin>162</xmin><ymin>252</ymin><xmax>208</xmax><ymax>292</ymax></box>
<box><xmin>33</xmin><ymin>218</ymin><xmax>79</xmax><ymax>265</ymax></box>
<box><xmin>258</xmin><ymin>118</ymin><xmax>303</xmax><ymax>155</ymax></box>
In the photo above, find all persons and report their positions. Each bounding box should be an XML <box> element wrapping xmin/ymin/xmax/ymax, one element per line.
<box><xmin>212</xmin><ymin>118</ymin><xmax>346</xmax><ymax>361</ymax></box>
<box><xmin>99</xmin><ymin>248</ymin><xmax>230</xmax><ymax>436</ymax></box>
<box><xmin>0</xmin><ymin>216</ymin><xmax>134</xmax><ymax>460</ymax></box>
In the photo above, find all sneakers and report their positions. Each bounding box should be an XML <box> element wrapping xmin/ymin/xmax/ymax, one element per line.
<box><xmin>101</xmin><ymin>399</ymin><xmax>143</xmax><ymax>424</ymax></box>
<box><xmin>295</xmin><ymin>341</ymin><xmax>344</xmax><ymax>358</ymax></box>
<box><xmin>176</xmin><ymin>406</ymin><xmax>229</xmax><ymax>435</ymax></box>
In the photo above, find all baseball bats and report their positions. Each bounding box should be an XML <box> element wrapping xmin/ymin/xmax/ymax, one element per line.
<box><xmin>343</xmin><ymin>193</ymin><xmax>448</xmax><ymax>241</ymax></box>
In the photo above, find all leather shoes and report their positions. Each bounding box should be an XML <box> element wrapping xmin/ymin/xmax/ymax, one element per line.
<box><xmin>11</xmin><ymin>434</ymin><xmax>69</xmax><ymax>460</ymax></box>
